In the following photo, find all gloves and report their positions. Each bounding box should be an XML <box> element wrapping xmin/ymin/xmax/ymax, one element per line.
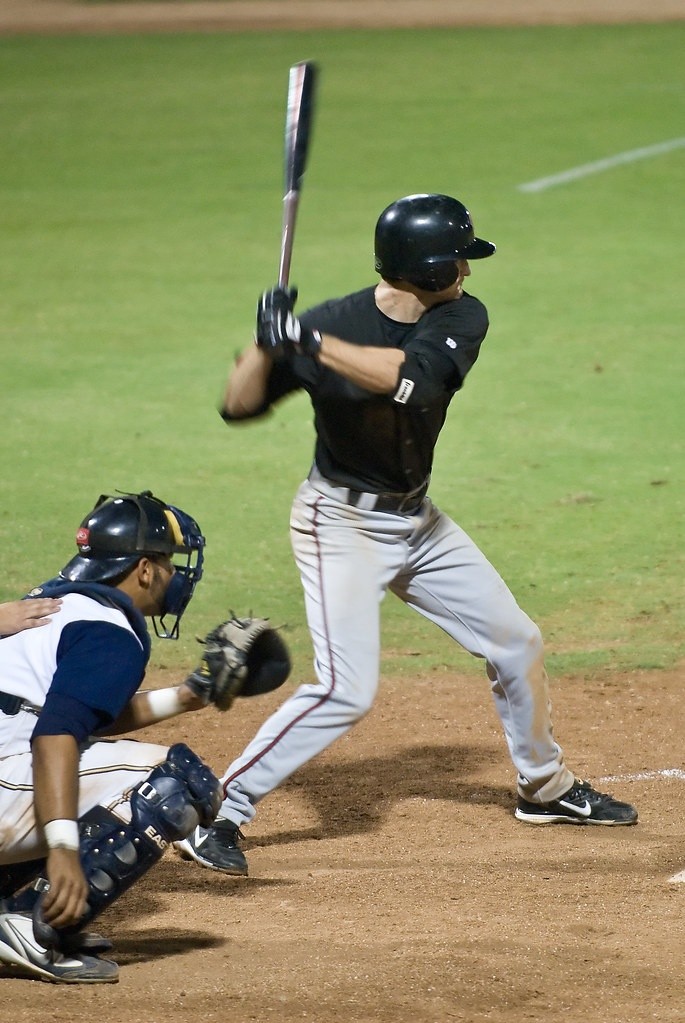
<box><xmin>253</xmin><ymin>284</ymin><xmax>323</xmax><ymax>360</ymax></box>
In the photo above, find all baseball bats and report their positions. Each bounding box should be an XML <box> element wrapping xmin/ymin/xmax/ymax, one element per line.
<box><xmin>280</xmin><ymin>59</ymin><xmax>318</xmax><ymax>290</ymax></box>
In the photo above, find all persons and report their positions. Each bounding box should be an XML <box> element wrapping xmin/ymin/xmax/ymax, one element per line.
<box><xmin>172</xmin><ymin>195</ymin><xmax>636</xmax><ymax>879</ymax></box>
<box><xmin>0</xmin><ymin>489</ymin><xmax>226</xmax><ymax>983</ymax></box>
<box><xmin>0</xmin><ymin>591</ymin><xmax>65</xmax><ymax>647</ymax></box>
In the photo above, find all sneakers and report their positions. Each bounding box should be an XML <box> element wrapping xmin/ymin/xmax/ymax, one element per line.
<box><xmin>172</xmin><ymin>823</ymin><xmax>249</xmax><ymax>876</ymax></box>
<box><xmin>514</xmin><ymin>777</ymin><xmax>638</xmax><ymax>824</ymax></box>
<box><xmin>0</xmin><ymin>898</ymin><xmax>120</xmax><ymax>984</ymax></box>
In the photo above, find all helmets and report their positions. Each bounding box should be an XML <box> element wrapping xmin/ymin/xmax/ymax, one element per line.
<box><xmin>59</xmin><ymin>495</ymin><xmax>178</xmax><ymax>582</ymax></box>
<box><xmin>374</xmin><ymin>193</ymin><xmax>497</xmax><ymax>292</ymax></box>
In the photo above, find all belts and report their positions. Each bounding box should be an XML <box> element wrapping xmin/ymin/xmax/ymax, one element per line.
<box><xmin>348</xmin><ymin>485</ymin><xmax>428</xmax><ymax>513</ymax></box>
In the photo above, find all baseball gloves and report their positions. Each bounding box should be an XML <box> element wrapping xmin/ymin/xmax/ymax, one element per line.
<box><xmin>189</xmin><ymin>607</ymin><xmax>292</xmax><ymax>712</ymax></box>
